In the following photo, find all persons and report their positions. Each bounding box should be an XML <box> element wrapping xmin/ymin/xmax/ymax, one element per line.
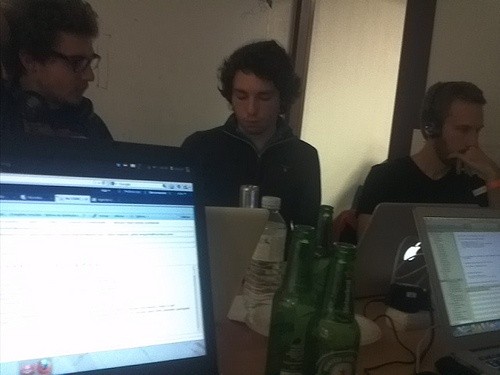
<box><xmin>337</xmin><ymin>81</ymin><xmax>500</xmax><ymax>246</ymax></box>
<box><xmin>160</xmin><ymin>40</ymin><xmax>321</xmax><ymax>228</ymax></box>
<box><xmin>0</xmin><ymin>0</ymin><xmax>122</xmax><ymax>142</ymax></box>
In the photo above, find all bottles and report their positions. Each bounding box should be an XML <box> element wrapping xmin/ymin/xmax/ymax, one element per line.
<box><xmin>243</xmin><ymin>196</ymin><xmax>287</xmax><ymax>313</ymax></box>
<box><xmin>266</xmin><ymin>224</ymin><xmax>321</xmax><ymax>375</ymax></box>
<box><xmin>311</xmin><ymin>205</ymin><xmax>335</xmax><ymax>291</ymax></box>
<box><xmin>309</xmin><ymin>242</ymin><xmax>361</xmax><ymax>375</ymax></box>
<box><xmin>239</xmin><ymin>184</ymin><xmax>262</xmax><ymax>209</ymax></box>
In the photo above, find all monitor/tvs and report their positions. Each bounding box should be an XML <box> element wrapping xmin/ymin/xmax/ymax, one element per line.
<box><xmin>0</xmin><ymin>128</ymin><xmax>219</xmax><ymax>375</ymax></box>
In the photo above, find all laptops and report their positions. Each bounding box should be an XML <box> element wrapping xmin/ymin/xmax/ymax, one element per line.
<box><xmin>352</xmin><ymin>203</ymin><xmax>439</xmax><ymax>299</ymax></box>
<box><xmin>203</xmin><ymin>205</ymin><xmax>271</xmax><ymax>322</ymax></box>
<box><xmin>412</xmin><ymin>206</ymin><xmax>500</xmax><ymax>375</ymax></box>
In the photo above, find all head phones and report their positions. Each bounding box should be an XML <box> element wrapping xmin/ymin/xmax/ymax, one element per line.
<box><xmin>423</xmin><ymin>82</ymin><xmax>441</xmax><ymax>139</ymax></box>
<box><xmin>220</xmin><ymin>64</ymin><xmax>301</xmax><ymax>106</ymax></box>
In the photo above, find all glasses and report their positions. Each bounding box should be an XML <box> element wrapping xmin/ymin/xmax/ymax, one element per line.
<box><xmin>46</xmin><ymin>46</ymin><xmax>101</xmax><ymax>74</ymax></box>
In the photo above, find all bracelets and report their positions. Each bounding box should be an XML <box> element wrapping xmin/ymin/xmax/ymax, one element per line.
<box><xmin>488</xmin><ymin>180</ymin><xmax>499</xmax><ymax>189</ymax></box>
